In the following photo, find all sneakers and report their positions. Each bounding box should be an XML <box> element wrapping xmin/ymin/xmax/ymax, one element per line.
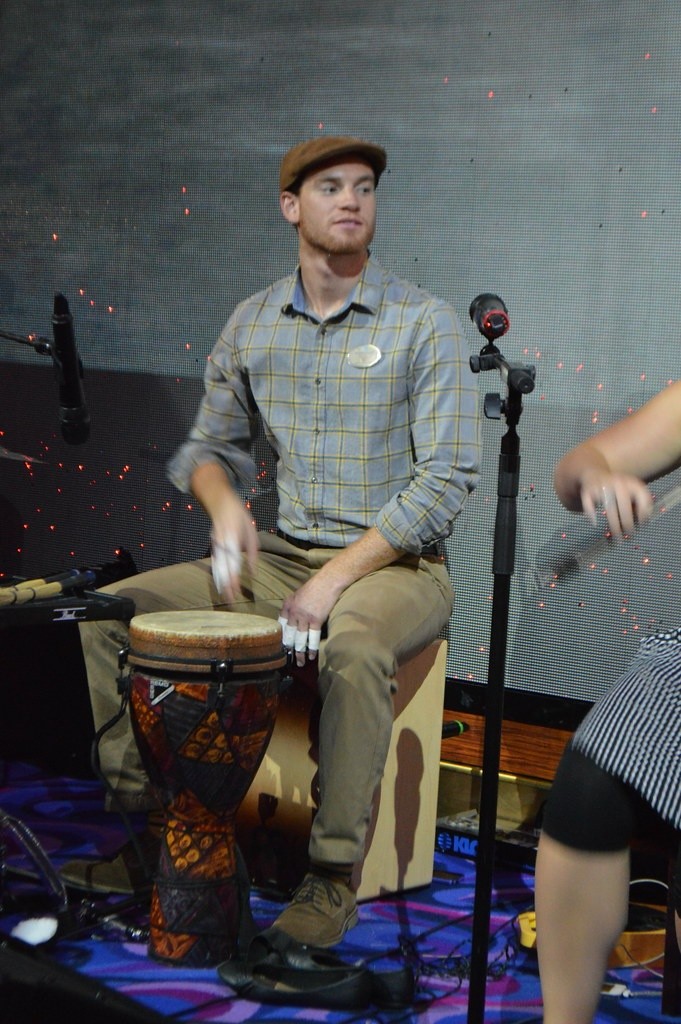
<box><xmin>270</xmin><ymin>866</ymin><xmax>360</xmax><ymax>949</ymax></box>
<box><xmin>54</xmin><ymin>829</ymin><xmax>161</xmax><ymax>895</ymax></box>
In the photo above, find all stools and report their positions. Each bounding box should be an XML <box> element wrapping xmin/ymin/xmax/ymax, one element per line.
<box><xmin>231</xmin><ymin>638</ymin><xmax>447</xmax><ymax>901</ymax></box>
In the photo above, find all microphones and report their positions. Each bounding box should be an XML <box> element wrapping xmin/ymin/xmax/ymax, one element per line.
<box><xmin>52</xmin><ymin>292</ymin><xmax>91</xmax><ymax>445</ymax></box>
<box><xmin>469</xmin><ymin>293</ymin><xmax>510</xmax><ymax>338</ymax></box>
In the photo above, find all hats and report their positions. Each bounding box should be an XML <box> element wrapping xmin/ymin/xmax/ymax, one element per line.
<box><xmin>279</xmin><ymin>136</ymin><xmax>388</xmax><ymax>193</ymax></box>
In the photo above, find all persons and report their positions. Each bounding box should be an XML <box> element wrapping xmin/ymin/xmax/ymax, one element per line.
<box><xmin>535</xmin><ymin>380</ymin><xmax>681</xmax><ymax>1024</ymax></box>
<box><xmin>58</xmin><ymin>137</ymin><xmax>485</xmax><ymax>948</ymax></box>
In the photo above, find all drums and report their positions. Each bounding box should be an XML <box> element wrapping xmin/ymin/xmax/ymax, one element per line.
<box><xmin>124</xmin><ymin>608</ymin><xmax>291</xmax><ymax>970</ymax></box>
